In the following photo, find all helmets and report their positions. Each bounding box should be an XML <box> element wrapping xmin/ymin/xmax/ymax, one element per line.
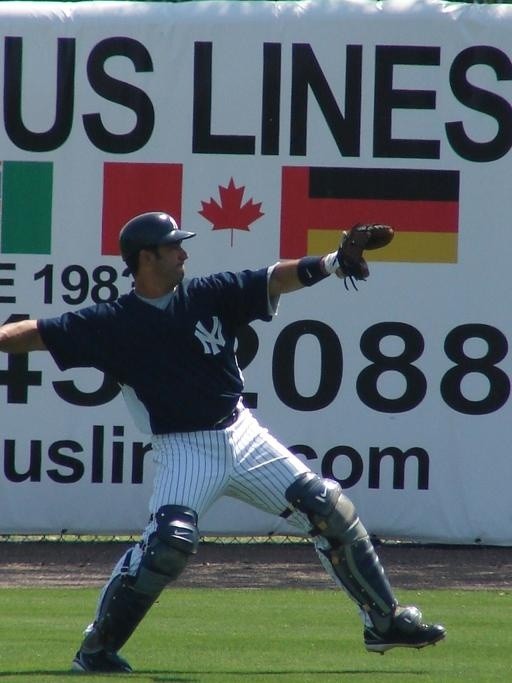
<box><xmin>119</xmin><ymin>211</ymin><xmax>196</xmax><ymax>260</ymax></box>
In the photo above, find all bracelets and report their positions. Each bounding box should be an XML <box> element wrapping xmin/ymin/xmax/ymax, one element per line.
<box><xmin>296</xmin><ymin>256</ymin><xmax>328</xmax><ymax>285</ymax></box>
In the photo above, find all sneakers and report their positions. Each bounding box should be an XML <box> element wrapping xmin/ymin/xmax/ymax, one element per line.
<box><xmin>72</xmin><ymin>650</ymin><xmax>132</xmax><ymax>672</ymax></box>
<box><xmin>364</xmin><ymin>622</ymin><xmax>448</xmax><ymax>654</ymax></box>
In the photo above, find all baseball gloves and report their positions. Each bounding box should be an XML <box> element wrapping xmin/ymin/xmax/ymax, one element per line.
<box><xmin>333</xmin><ymin>223</ymin><xmax>394</xmax><ymax>291</ymax></box>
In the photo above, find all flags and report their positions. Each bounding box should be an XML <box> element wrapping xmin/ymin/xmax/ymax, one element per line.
<box><xmin>1</xmin><ymin>161</ymin><xmax>309</xmax><ymax>265</ymax></box>
<box><xmin>310</xmin><ymin>167</ymin><xmax>459</xmax><ymax>263</ymax></box>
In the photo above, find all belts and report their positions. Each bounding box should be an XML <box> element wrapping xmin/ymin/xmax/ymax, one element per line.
<box><xmin>205</xmin><ymin>410</ymin><xmax>237</xmax><ymax>429</ymax></box>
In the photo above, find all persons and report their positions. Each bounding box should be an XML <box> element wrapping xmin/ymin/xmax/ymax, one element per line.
<box><xmin>0</xmin><ymin>211</ymin><xmax>448</xmax><ymax>673</ymax></box>
